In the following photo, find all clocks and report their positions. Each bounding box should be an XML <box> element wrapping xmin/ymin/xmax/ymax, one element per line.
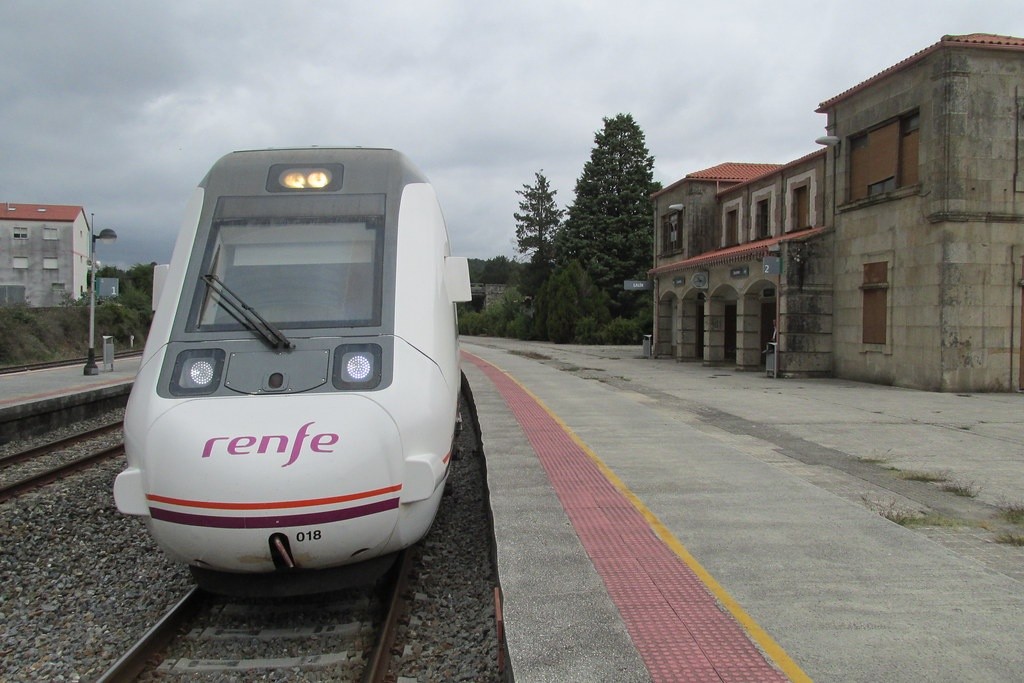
<box><xmin>692</xmin><ymin>271</ymin><xmax>709</xmax><ymax>289</ymax></box>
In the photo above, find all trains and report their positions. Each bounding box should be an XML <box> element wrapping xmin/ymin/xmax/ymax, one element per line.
<box><xmin>112</xmin><ymin>142</ymin><xmax>477</xmax><ymax>595</ymax></box>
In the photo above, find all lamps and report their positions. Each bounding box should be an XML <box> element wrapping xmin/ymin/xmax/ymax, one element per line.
<box><xmin>815</xmin><ymin>136</ymin><xmax>841</xmax><ymax>146</ymax></box>
<box><xmin>669</xmin><ymin>204</ymin><xmax>686</xmax><ymax>210</ymax></box>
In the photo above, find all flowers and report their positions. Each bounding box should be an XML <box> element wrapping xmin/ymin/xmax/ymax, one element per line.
<box><xmin>791</xmin><ymin>245</ymin><xmax>814</xmax><ymax>263</ymax></box>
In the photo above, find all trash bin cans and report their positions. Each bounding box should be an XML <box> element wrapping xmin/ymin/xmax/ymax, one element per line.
<box><xmin>642</xmin><ymin>335</ymin><xmax>652</xmax><ymax>360</ymax></box>
<box><xmin>765</xmin><ymin>342</ymin><xmax>776</xmax><ymax>378</ymax></box>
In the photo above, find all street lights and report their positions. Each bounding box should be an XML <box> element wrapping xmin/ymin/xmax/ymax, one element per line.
<box><xmin>83</xmin><ymin>228</ymin><xmax>118</xmax><ymax>375</ymax></box>
